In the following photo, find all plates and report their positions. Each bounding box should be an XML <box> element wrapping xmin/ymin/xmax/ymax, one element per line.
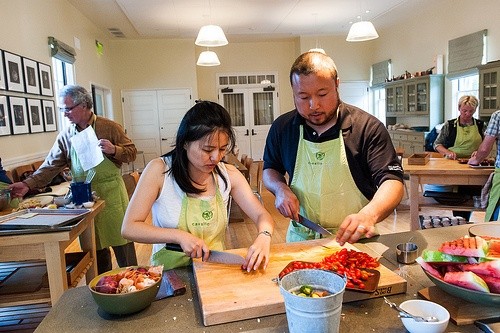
<box><xmin>471</xmin><ymin>166</ymin><xmax>495</xmax><ymax>169</ymax></box>
<box><xmin>421</xmin><ymin>266</ymin><xmax>500</xmax><ymax>308</ymax></box>
<box><xmin>278</xmin><ymin>261</ymin><xmax>380</xmax><ymax>293</ymax></box>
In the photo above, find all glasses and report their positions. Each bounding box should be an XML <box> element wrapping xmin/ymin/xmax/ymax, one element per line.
<box><xmin>58</xmin><ymin>103</ymin><xmax>80</xmax><ymax>113</ymax></box>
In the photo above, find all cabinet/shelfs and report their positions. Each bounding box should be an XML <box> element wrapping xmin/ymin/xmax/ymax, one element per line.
<box><xmin>476</xmin><ymin>60</ymin><xmax>500</xmax><ymax>116</ymax></box>
<box><xmin>384</xmin><ymin>74</ymin><xmax>446</xmax><ymax>158</ymax></box>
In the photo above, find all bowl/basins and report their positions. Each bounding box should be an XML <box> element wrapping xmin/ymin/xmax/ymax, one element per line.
<box><xmin>399</xmin><ymin>299</ymin><xmax>450</xmax><ymax>333</ymax></box>
<box><xmin>458</xmin><ymin>159</ymin><xmax>468</xmax><ymax>163</ymax></box>
<box><xmin>82</xmin><ymin>202</ymin><xmax>95</xmax><ymax>208</ymax></box>
<box><xmin>54</xmin><ymin>198</ymin><xmax>73</xmax><ymax>206</ymax></box>
<box><xmin>88</xmin><ymin>265</ymin><xmax>162</xmax><ymax>314</ymax></box>
<box><xmin>434</xmin><ymin>192</ymin><xmax>468</xmax><ymax>205</ymax></box>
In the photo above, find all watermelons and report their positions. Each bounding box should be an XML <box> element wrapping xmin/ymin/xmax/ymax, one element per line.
<box><xmin>415</xmin><ymin>234</ymin><xmax>500</xmax><ymax>295</ymax></box>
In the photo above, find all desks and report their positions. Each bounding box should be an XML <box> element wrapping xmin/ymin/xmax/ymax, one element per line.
<box><xmin>401</xmin><ymin>157</ymin><xmax>496</xmax><ymax>231</ymax></box>
<box><xmin>0</xmin><ymin>199</ymin><xmax>106</xmax><ymax>308</ymax></box>
<box><xmin>220</xmin><ymin>148</ymin><xmax>248</xmax><ymax>223</ymax></box>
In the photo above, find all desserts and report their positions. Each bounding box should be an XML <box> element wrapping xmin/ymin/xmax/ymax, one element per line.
<box><xmin>422</xmin><ymin>215</ymin><xmax>466</xmax><ymax>228</ymax></box>
<box><xmin>472</xmin><ymin>159</ymin><xmax>495</xmax><ymax>167</ymax></box>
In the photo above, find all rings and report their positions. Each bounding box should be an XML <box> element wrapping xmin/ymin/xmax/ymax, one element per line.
<box><xmin>100</xmin><ymin>145</ymin><xmax>103</xmax><ymax>148</ymax></box>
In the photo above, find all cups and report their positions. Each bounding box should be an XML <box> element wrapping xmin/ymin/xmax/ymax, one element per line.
<box><xmin>473</xmin><ymin>196</ymin><xmax>481</xmax><ymax>207</ymax></box>
<box><xmin>397</xmin><ymin>243</ymin><xmax>418</xmax><ymax>264</ymax></box>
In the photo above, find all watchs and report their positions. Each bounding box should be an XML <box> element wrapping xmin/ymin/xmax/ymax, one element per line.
<box><xmin>257</xmin><ymin>231</ymin><xmax>272</xmax><ymax>239</ymax></box>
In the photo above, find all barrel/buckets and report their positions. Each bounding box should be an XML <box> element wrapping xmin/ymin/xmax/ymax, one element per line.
<box><xmin>70</xmin><ymin>182</ymin><xmax>93</xmax><ymax>205</ymax></box>
<box><xmin>276</xmin><ymin>267</ymin><xmax>348</xmax><ymax>333</ymax></box>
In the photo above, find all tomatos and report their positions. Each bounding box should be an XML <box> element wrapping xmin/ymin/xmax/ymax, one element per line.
<box><xmin>281</xmin><ymin>248</ymin><xmax>379</xmax><ymax>287</ymax></box>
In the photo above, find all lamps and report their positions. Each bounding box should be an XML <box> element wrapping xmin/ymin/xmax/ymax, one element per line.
<box><xmin>346</xmin><ymin>15</ymin><xmax>379</xmax><ymax>42</ymax></box>
<box><xmin>308</xmin><ymin>35</ymin><xmax>326</xmax><ymax>55</ymax></box>
<box><xmin>195</xmin><ymin>25</ymin><xmax>228</xmax><ymax>47</ymax></box>
<box><xmin>196</xmin><ymin>47</ymin><xmax>221</xmax><ymax>66</ymax></box>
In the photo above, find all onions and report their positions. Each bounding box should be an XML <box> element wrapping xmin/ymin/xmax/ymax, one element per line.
<box><xmin>95</xmin><ymin>275</ymin><xmax>119</xmax><ymax>294</ymax></box>
<box><xmin>135</xmin><ymin>267</ymin><xmax>148</xmax><ymax>272</ymax></box>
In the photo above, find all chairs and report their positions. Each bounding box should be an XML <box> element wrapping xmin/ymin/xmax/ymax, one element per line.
<box><xmin>235</xmin><ymin>149</ymin><xmax>264</xmax><ymax>207</ymax></box>
<box><xmin>6</xmin><ymin>161</ymin><xmax>73</xmax><ymax>208</ymax></box>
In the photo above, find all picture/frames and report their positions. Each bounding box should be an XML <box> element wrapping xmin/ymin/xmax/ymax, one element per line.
<box><xmin>0</xmin><ymin>49</ymin><xmax>58</xmax><ymax>137</ymax></box>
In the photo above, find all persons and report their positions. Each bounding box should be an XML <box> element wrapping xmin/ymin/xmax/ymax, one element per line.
<box><xmin>0</xmin><ymin>158</ymin><xmax>22</xmax><ymax>211</ymax></box>
<box><xmin>432</xmin><ymin>95</ymin><xmax>488</xmax><ymax>163</ymax></box>
<box><xmin>469</xmin><ymin>110</ymin><xmax>500</xmax><ymax>222</ymax></box>
<box><xmin>122</xmin><ymin>100</ymin><xmax>273</xmax><ymax>274</ymax></box>
<box><xmin>8</xmin><ymin>85</ymin><xmax>137</xmax><ymax>277</ymax></box>
<box><xmin>261</xmin><ymin>52</ymin><xmax>404</xmax><ymax>245</ymax></box>
<box><xmin>51</xmin><ymin>165</ymin><xmax>72</xmax><ymax>185</ymax></box>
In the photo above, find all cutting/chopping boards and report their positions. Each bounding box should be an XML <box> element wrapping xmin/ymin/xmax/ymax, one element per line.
<box><xmin>191</xmin><ymin>236</ymin><xmax>407</xmax><ymax>326</ymax></box>
<box><xmin>416</xmin><ymin>285</ymin><xmax>500</xmax><ymax>326</ymax></box>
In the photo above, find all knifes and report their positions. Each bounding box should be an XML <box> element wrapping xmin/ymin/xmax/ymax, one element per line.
<box><xmin>165</xmin><ymin>243</ymin><xmax>246</xmax><ymax>265</ymax></box>
<box><xmin>298</xmin><ymin>214</ymin><xmax>334</xmax><ymax>236</ymax></box>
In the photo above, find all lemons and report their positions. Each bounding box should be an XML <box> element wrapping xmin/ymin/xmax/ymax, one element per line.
<box><xmin>48</xmin><ymin>204</ymin><xmax>57</xmax><ymax>209</ymax></box>
